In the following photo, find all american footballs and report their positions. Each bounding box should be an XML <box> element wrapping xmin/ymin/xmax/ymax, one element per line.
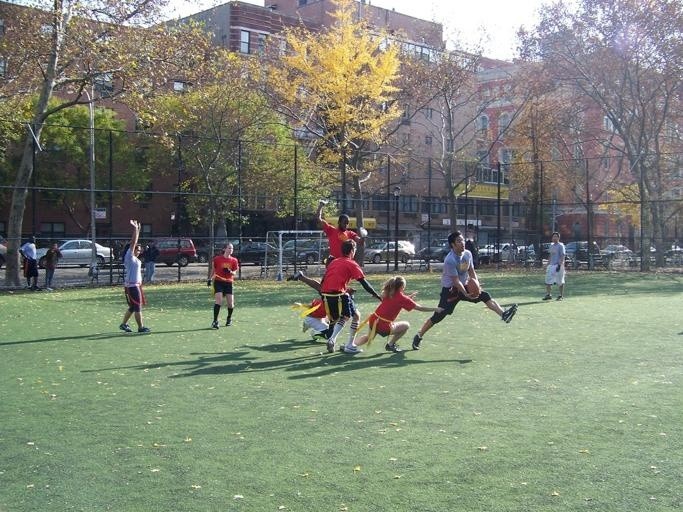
<box><xmin>467</xmin><ymin>277</ymin><xmax>481</xmax><ymax>298</ymax></box>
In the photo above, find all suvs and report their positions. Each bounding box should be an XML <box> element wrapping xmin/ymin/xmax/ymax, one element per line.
<box><xmin>566</xmin><ymin>241</ymin><xmax>603</xmax><ymax>266</ymax></box>
<box><xmin>416</xmin><ymin>239</ymin><xmax>478</xmax><ymax>263</ymax></box>
<box><xmin>140</xmin><ymin>237</ymin><xmax>196</xmax><ymax>267</ymax></box>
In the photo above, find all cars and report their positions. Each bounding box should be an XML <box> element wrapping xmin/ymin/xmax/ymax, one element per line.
<box><xmin>197</xmin><ymin>239</ymin><xmax>240</xmax><ymax>262</ymax></box>
<box><xmin>20</xmin><ymin>239</ymin><xmax>114</xmax><ymax>269</ymax></box>
<box><xmin>229</xmin><ymin>239</ymin><xmax>278</xmax><ymax>266</ymax></box>
<box><xmin>478</xmin><ymin>242</ymin><xmax>551</xmax><ymax>264</ymax></box>
<box><xmin>281</xmin><ymin>239</ymin><xmax>331</xmax><ymax>265</ymax></box>
<box><xmin>0</xmin><ymin>234</ymin><xmax>8</xmax><ymax>269</ymax></box>
<box><xmin>599</xmin><ymin>245</ymin><xmax>633</xmax><ymax>261</ymax></box>
<box><xmin>362</xmin><ymin>241</ymin><xmax>413</xmax><ymax>264</ymax></box>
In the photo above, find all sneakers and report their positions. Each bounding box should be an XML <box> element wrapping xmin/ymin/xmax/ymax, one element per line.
<box><xmin>212</xmin><ymin>321</ymin><xmax>219</xmax><ymax>329</ymax></box>
<box><xmin>543</xmin><ymin>295</ymin><xmax>552</xmax><ymax>300</ymax></box>
<box><xmin>303</xmin><ymin>319</ymin><xmax>312</xmax><ymax>333</ymax></box>
<box><xmin>502</xmin><ymin>308</ymin><xmax>517</xmax><ymax>323</ymax></box>
<box><xmin>340</xmin><ymin>343</ymin><xmax>363</xmax><ymax>353</ymax></box>
<box><xmin>557</xmin><ymin>296</ymin><xmax>562</xmax><ymax>300</ymax></box>
<box><xmin>314</xmin><ymin>333</ymin><xmax>329</xmax><ymax>341</ymax></box>
<box><xmin>120</xmin><ymin>324</ymin><xmax>132</xmax><ymax>332</ymax></box>
<box><xmin>226</xmin><ymin>320</ymin><xmax>231</xmax><ymax>326</ymax></box>
<box><xmin>326</xmin><ymin>338</ymin><xmax>335</xmax><ymax>354</ymax></box>
<box><xmin>138</xmin><ymin>328</ymin><xmax>150</xmax><ymax>332</ymax></box>
<box><xmin>385</xmin><ymin>343</ymin><xmax>402</xmax><ymax>353</ymax></box>
<box><xmin>286</xmin><ymin>271</ymin><xmax>303</xmax><ymax>281</ymax></box>
<box><xmin>412</xmin><ymin>335</ymin><xmax>423</xmax><ymax>350</ymax></box>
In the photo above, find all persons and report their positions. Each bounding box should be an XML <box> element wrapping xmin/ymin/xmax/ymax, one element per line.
<box><xmin>465</xmin><ymin>239</ymin><xmax>534</xmax><ymax>269</ymax></box>
<box><xmin>293</xmin><ymin>199</ymin><xmax>445</xmax><ymax>354</ymax></box>
<box><xmin>19</xmin><ymin>235</ymin><xmax>38</xmax><ymax>290</ymax></box>
<box><xmin>143</xmin><ymin>240</ymin><xmax>160</xmax><ymax>282</ymax></box>
<box><xmin>119</xmin><ymin>220</ymin><xmax>150</xmax><ymax>332</ymax></box>
<box><xmin>412</xmin><ymin>232</ymin><xmax>519</xmax><ymax>349</ymax></box>
<box><xmin>46</xmin><ymin>243</ymin><xmax>63</xmax><ymax>290</ymax></box>
<box><xmin>542</xmin><ymin>232</ymin><xmax>565</xmax><ymax>301</ymax></box>
<box><xmin>207</xmin><ymin>243</ymin><xmax>238</xmax><ymax>328</ymax></box>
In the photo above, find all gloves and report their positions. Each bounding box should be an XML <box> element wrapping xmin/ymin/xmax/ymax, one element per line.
<box><xmin>223</xmin><ymin>268</ymin><xmax>230</xmax><ymax>273</ymax></box>
<box><xmin>208</xmin><ymin>279</ymin><xmax>211</xmax><ymax>286</ymax></box>
<box><xmin>556</xmin><ymin>265</ymin><xmax>560</xmax><ymax>272</ymax></box>
